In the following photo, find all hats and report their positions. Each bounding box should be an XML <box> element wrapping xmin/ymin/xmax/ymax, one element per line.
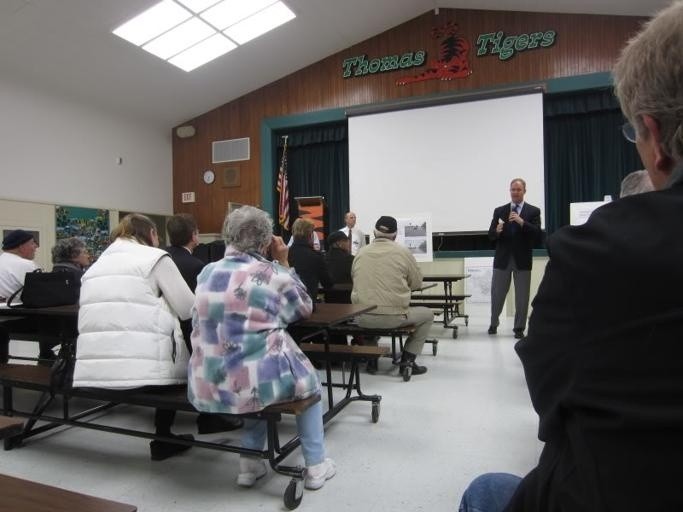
<box><xmin>375</xmin><ymin>216</ymin><xmax>397</xmax><ymax>233</ymax></box>
<box><xmin>3</xmin><ymin>229</ymin><xmax>32</xmax><ymax>249</ymax></box>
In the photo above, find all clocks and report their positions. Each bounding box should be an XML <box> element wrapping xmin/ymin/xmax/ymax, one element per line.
<box><xmin>203</xmin><ymin>169</ymin><xmax>215</xmax><ymax>185</ymax></box>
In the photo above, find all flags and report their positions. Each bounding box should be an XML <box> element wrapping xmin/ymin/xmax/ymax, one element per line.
<box><xmin>277</xmin><ymin>144</ymin><xmax>291</xmax><ymax>231</ymax></box>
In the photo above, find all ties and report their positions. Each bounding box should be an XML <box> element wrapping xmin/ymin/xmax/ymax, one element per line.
<box><xmin>512</xmin><ymin>204</ymin><xmax>520</xmax><ymax>213</ymax></box>
<box><xmin>348</xmin><ymin>230</ymin><xmax>351</xmax><ymax>253</ymax></box>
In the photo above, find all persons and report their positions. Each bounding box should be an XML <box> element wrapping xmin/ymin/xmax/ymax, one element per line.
<box><xmin>488</xmin><ymin>179</ymin><xmax>541</xmax><ymax>339</ymax></box>
<box><xmin>459</xmin><ymin>1</ymin><xmax>683</xmax><ymax>512</ymax></box>
<box><xmin>619</xmin><ymin>170</ymin><xmax>656</xmax><ymax>199</ymax></box>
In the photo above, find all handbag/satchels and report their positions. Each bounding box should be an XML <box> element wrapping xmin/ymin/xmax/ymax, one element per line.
<box><xmin>51</xmin><ymin>344</ymin><xmax>76</xmax><ymax>392</ymax></box>
<box><xmin>21</xmin><ymin>270</ymin><xmax>79</xmax><ymax>308</ymax></box>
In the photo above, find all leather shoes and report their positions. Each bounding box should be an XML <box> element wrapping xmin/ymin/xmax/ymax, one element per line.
<box><xmin>488</xmin><ymin>326</ymin><xmax>496</xmax><ymax>333</ymax></box>
<box><xmin>401</xmin><ymin>360</ymin><xmax>426</xmax><ymax>375</ymax></box>
<box><xmin>513</xmin><ymin>328</ymin><xmax>524</xmax><ymax>338</ymax></box>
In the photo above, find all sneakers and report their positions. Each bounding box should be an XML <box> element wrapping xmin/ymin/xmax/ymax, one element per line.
<box><xmin>305</xmin><ymin>458</ymin><xmax>335</xmax><ymax>489</ymax></box>
<box><xmin>150</xmin><ymin>434</ymin><xmax>193</xmax><ymax>460</ymax></box>
<box><xmin>238</xmin><ymin>455</ymin><xmax>266</xmax><ymax>487</ymax></box>
<box><xmin>196</xmin><ymin>413</ymin><xmax>241</xmax><ymax>433</ymax></box>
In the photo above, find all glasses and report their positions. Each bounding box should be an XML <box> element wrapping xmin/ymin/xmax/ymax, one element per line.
<box><xmin>622</xmin><ymin>120</ymin><xmax>637</xmax><ymax>142</ymax></box>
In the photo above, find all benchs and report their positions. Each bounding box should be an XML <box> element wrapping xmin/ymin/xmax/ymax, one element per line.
<box><xmin>0</xmin><ymin>261</ymin><xmax>473</xmax><ymax>372</ymax></box>
<box><xmin>0</xmin><ymin>301</ymin><xmax>390</xmax><ymax>512</ymax></box>
